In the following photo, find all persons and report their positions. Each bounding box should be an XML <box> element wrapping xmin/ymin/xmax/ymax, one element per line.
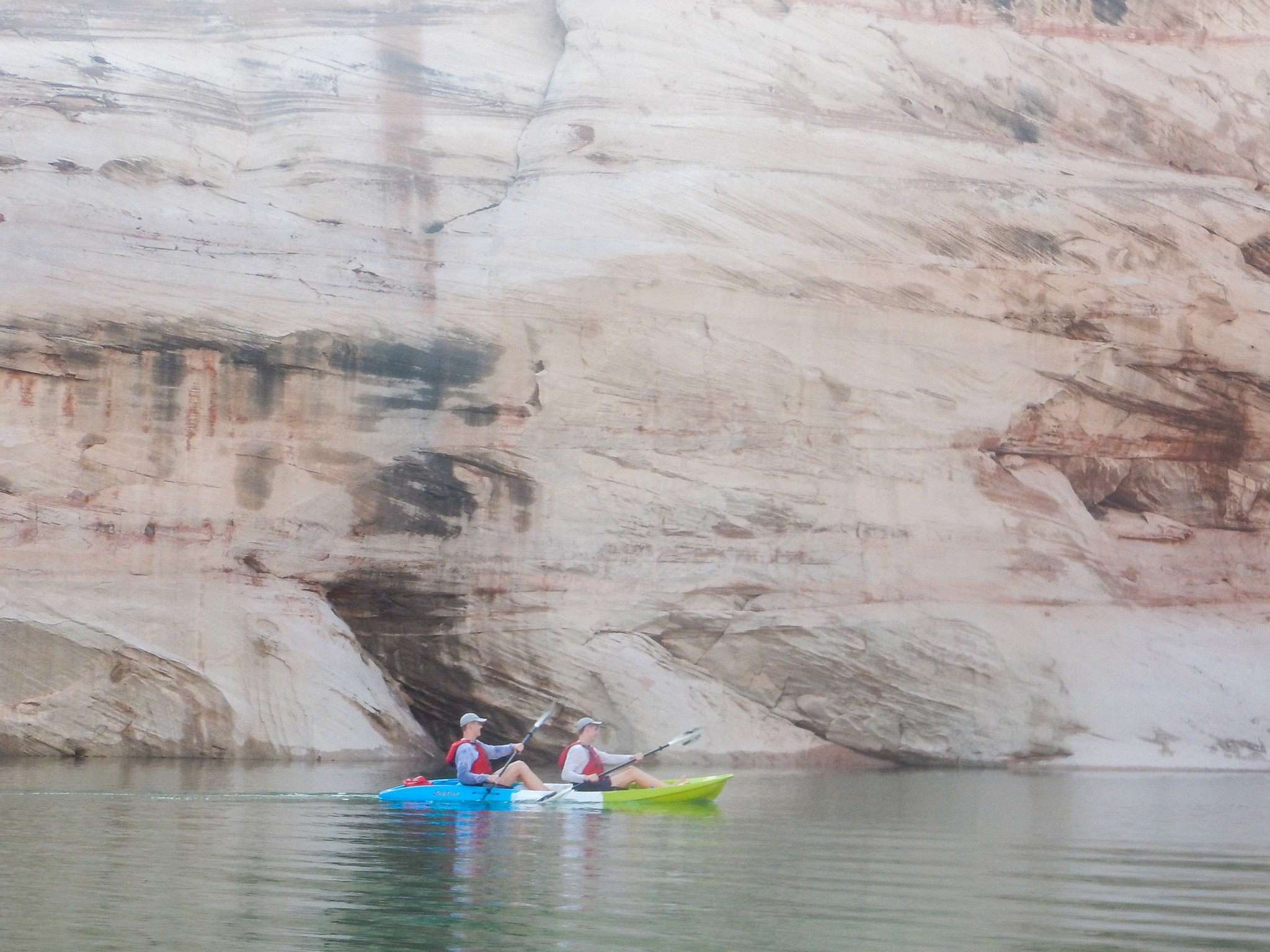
<box><xmin>446</xmin><ymin>713</ymin><xmax>552</xmax><ymax>791</ymax></box>
<box><xmin>557</xmin><ymin>717</ymin><xmax>690</xmax><ymax>792</ymax></box>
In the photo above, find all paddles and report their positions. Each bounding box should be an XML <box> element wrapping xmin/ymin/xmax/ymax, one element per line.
<box><xmin>538</xmin><ymin>725</ymin><xmax>704</xmax><ymax>803</ymax></box>
<box><xmin>480</xmin><ymin>698</ymin><xmax>558</xmax><ymax>802</ymax></box>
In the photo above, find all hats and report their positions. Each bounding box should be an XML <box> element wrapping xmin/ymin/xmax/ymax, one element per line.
<box><xmin>460</xmin><ymin>713</ymin><xmax>487</xmax><ymax>727</ymax></box>
<box><xmin>577</xmin><ymin>717</ymin><xmax>602</xmax><ymax>731</ymax></box>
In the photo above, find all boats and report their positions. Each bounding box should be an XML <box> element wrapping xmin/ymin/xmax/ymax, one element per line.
<box><xmin>378</xmin><ymin>773</ymin><xmax>735</xmax><ymax>805</ymax></box>
